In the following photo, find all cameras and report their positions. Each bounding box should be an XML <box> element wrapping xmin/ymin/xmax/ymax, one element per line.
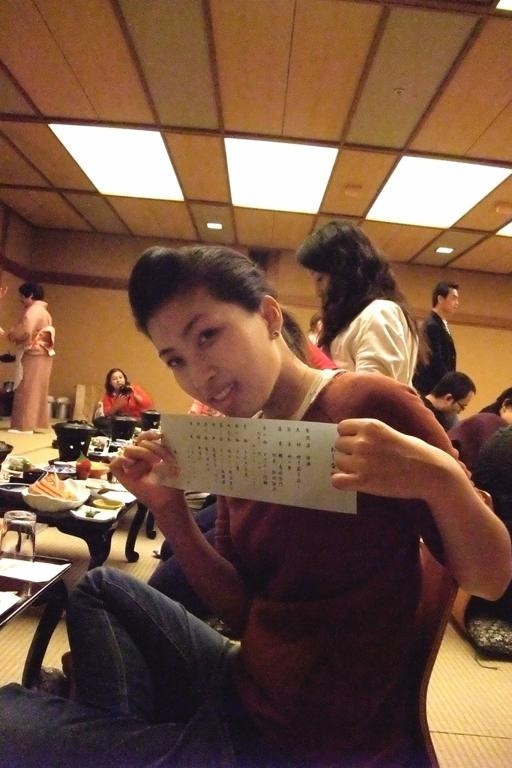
<box><xmin>120</xmin><ymin>385</ymin><xmax>132</xmax><ymax>395</ymax></box>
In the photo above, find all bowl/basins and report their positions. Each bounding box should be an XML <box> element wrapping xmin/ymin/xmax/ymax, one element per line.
<box><xmin>1</xmin><ymin>483</ymin><xmax>30</xmax><ymax>501</ymax></box>
<box><xmin>19</xmin><ymin>481</ymin><xmax>91</xmax><ymax>512</ymax></box>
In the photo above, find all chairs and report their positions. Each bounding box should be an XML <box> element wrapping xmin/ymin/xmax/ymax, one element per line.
<box><xmin>417</xmin><ymin>534</ymin><xmax>459</xmax><ymax>768</ymax></box>
<box><xmin>452</xmin><ymin>486</ymin><xmax>512</xmax><ymax>654</ymax></box>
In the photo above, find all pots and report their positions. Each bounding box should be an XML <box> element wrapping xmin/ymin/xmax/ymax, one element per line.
<box><xmin>51</xmin><ymin>420</ymin><xmax>98</xmax><ymax>441</ymax></box>
<box><xmin>139</xmin><ymin>409</ymin><xmax>160</xmax><ymax>420</ymax></box>
<box><xmin>108</xmin><ymin>414</ymin><xmax>136</xmax><ymax>427</ymax></box>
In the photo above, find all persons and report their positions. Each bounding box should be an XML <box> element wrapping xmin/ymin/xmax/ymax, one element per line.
<box><xmin>8</xmin><ymin>278</ymin><xmax>57</xmax><ymax>430</ymax></box>
<box><xmin>452</xmin><ymin>418</ymin><xmax>510</xmax><ymax>636</ymax></box>
<box><xmin>294</xmin><ymin>220</ymin><xmax>425</xmax><ymax>386</ymax></box>
<box><xmin>97</xmin><ymin>369</ymin><xmax>156</xmax><ymax>422</ymax></box>
<box><xmin>3</xmin><ymin>243</ymin><xmax>511</xmax><ymax>768</ymax></box>
<box><xmin>302</xmin><ymin>312</ymin><xmax>322</xmax><ymax>344</ymax></box>
<box><xmin>415</xmin><ymin>280</ymin><xmax>461</xmax><ymax>426</ymax></box>
<box><xmin>422</xmin><ymin>383</ymin><xmax>512</xmax><ymax>569</ymax></box>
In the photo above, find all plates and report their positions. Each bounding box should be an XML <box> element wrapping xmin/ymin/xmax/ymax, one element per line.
<box><xmin>69</xmin><ymin>505</ymin><xmax>124</xmax><ymax>524</ymax></box>
<box><xmin>86</xmin><ymin>476</ymin><xmax>138</xmax><ymax>509</ymax></box>
<box><xmin>37</xmin><ymin>464</ymin><xmax>77</xmax><ymax>475</ymax></box>
